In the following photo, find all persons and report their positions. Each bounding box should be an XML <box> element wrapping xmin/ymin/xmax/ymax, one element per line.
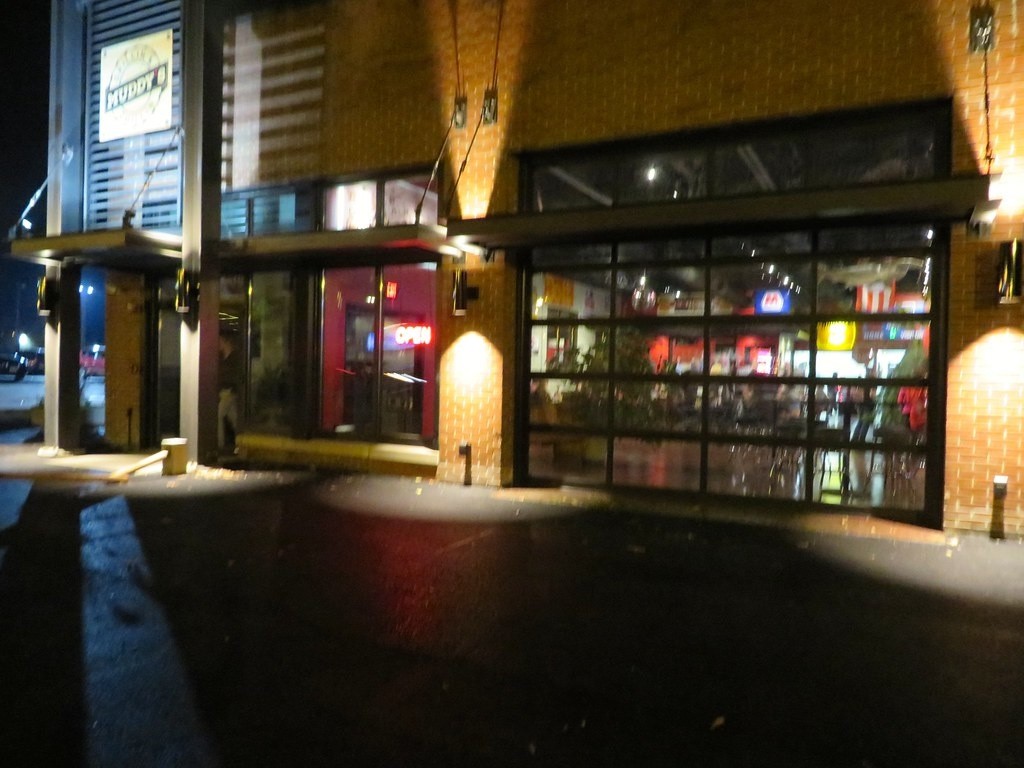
<box><xmin>218</xmin><ymin>330</ymin><xmax>238</xmax><ymax>454</ymax></box>
<box><xmin>851</xmin><ymin>369</ymin><xmax>877</xmax><ymax>442</ymax></box>
<box><xmin>814</xmin><ymin>383</ymin><xmax>827</xmax><ymax>422</ymax></box>
<box><xmin>826</xmin><ymin>372</ymin><xmax>838</xmax><ymax>415</ymax></box>
<box><xmin>838</xmin><ymin>385</ymin><xmax>847</xmax><ymax>416</ymax></box>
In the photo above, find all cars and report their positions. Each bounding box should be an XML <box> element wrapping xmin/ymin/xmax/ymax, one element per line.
<box><xmin>0</xmin><ymin>343</ymin><xmax>106</xmax><ymax>380</ymax></box>
